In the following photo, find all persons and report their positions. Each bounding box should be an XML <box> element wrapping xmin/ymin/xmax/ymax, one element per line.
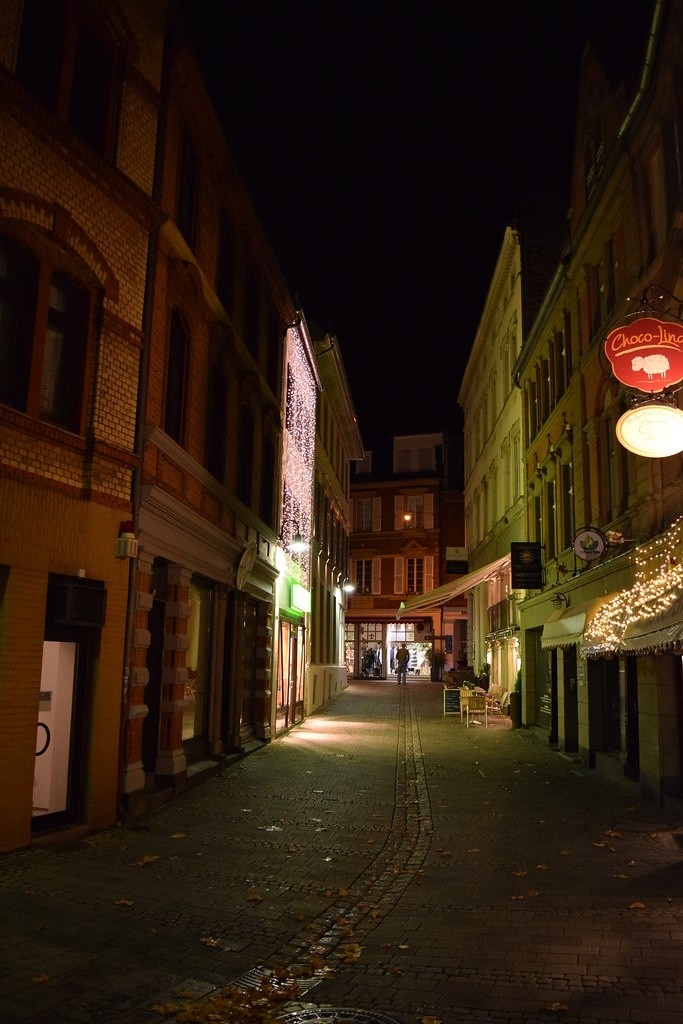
<box><xmin>396</xmin><ymin>643</ymin><xmax>410</xmax><ymax>685</ymax></box>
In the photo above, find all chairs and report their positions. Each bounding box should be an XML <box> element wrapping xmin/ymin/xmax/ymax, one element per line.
<box><xmin>466</xmin><ymin>696</ymin><xmax>489</xmax><ymax>728</ymax></box>
<box><xmin>487</xmin><ymin>692</ymin><xmax>508</xmax><ymax>724</ymax></box>
<box><xmin>460</xmin><ymin>690</ymin><xmax>474</xmax><ymax>724</ymax></box>
